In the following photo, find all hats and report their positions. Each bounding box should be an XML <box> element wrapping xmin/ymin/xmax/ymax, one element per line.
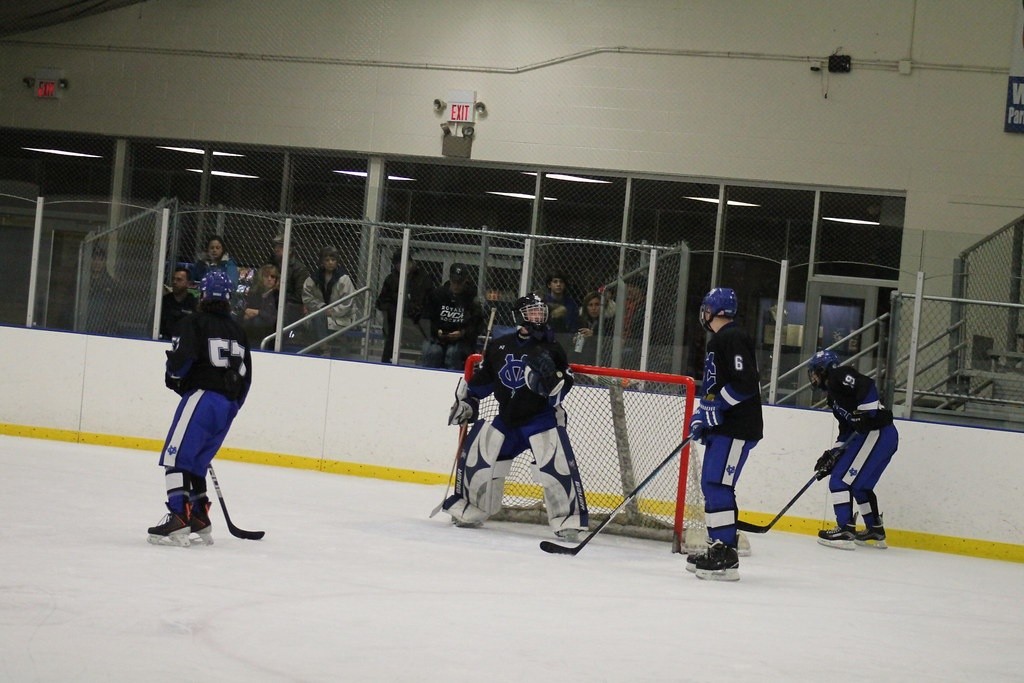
<box><xmin>274</xmin><ymin>234</ymin><xmax>297</xmax><ymax>245</ymax></box>
<box><xmin>391</xmin><ymin>248</ymin><xmax>412</xmax><ymax>265</ymax></box>
<box><xmin>450</xmin><ymin>263</ymin><xmax>466</xmax><ymax>282</ymax></box>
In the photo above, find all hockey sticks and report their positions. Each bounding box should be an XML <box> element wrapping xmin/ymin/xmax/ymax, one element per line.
<box><xmin>539</xmin><ymin>424</ymin><xmax>706</xmax><ymax>556</ymax></box>
<box><xmin>167</xmin><ymin>348</ymin><xmax>266</xmax><ymax>540</ymax></box>
<box><xmin>734</xmin><ymin>429</ymin><xmax>861</xmax><ymax>534</ymax></box>
<box><xmin>429</xmin><ymin>305</ymin><xmax>499</xmax><ymax>520</ymax></box>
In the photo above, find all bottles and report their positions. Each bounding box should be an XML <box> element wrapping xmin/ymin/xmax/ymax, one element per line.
<box><xmin>574</xmin><ymin>332</ymin><xmax>585</xmax><ymax>353</ymax></box>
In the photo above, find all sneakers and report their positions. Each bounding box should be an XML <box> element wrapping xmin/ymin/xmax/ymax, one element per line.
<box><xmin>854</xmin><ymin>512</ymin><xmax>887</xmax><ymax>548</ymax></box>
<box><xmin>188</xmin><ymin>495</ymin><xmax>214</xmax><ymax>546</ymax></box>
<box><xmin>146</xmin><ymin>495</ymin><xmax>191</xmax><ymax>546</ymax></box>
<box><xmin>695</xmin><ymin>534</ymin><xmax>740</xmax><ymax>581</ymax></box>
<box><xmin>816</xmin><ymin>511</ymin><xmax>859</xmax><ymax>550</ymax></box>
<box><xmin>686</xmin><ymin>551</ymin><xmax>709</xmax><ymax>573</ymax></box>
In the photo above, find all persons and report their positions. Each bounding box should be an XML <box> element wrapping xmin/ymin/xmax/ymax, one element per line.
<box><xmin>146</xmin><ymin>271</ymin><xmax>252</xmax><ymax>546</ymax></box>
<box><xmin>441</xmin><ymin>292</ymin><xmax>589</xmax><ymax>542</ymax></box>
<box><xmin>374</xmin><ymin>247</ymin><xmax>645</xmax><ymax>391</ymax></box>
<box><xmin>807</xmin><ymin>349</ymin><xmax>899</xmax><ymax>551</ymax></box>
<box><xmin>685</xmin><ymin>287</ymin><xmax>764</xmax><ymax>581</ymax></box>
<box><xmin>85</xmin><ymin>235</ymin><xmax>357</xmax><ymax>358</ymax></box>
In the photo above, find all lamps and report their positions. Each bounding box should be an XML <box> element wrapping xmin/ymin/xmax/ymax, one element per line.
<box><xmin>24</xmin><ymin>66</ymin><xmax>71</xmax><ymax>100</ymax></box>
<box><xmin>433</xmin><ymin>88</ymin><xmax>486</xmax><ymax>123</ymax></box>
<box><xmin>440</xmin><ymin>123</ymin><xmax>474</xmax><ymax>158</ymax></box>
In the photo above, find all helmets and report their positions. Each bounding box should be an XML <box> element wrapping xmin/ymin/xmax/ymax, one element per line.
<box><xmin>807</xmin><ymin>350</ymin><xmax>840</xmax><ymax>394</ymax></box>
<box><xmin>511</xmin><ymin>293</ymin><xmax>549</xmax><ymax>330</ymax></box>
<box><xmin>544</xmin><ymin>269</ymin><xmax>566</xmax><ymax>288</ymax></box>
<box><xmin>200</xmin><ymin>270</ymin><xmax>232</xmax><ymax>300</ymax></box>
<box><xmin>700</xmin><ymin>287</ymin><xmax>738</xmax><ymax>334</ymax></box>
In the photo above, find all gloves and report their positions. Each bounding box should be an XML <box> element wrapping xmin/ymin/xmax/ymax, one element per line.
<box><xmin>458</xmin><ymin>396</ymin><xmax>481</xmax><ymax>424</ymax></box>
<box><xmin>698</xmin><ymin>396</ymin><xmax>723</xmax><ymax>428</ymax></box>
<box><xmin>687</xmin><ymin>406</ymin><xmax>707</xmax><ymax>445</ymax></box>
<box><xmin>813</xmin><ymin>448</ymin><xmax>842</xmax><ymax>481</ymax></box>
<box><xmin>847</xmin><ymin>410</ymin><xmax>870</xmax><ymax>434</ymax></box>
<box><xmin>524</xmin><ymin>345</ymin><xmax>556</xmax><ymax>384</ymax></box>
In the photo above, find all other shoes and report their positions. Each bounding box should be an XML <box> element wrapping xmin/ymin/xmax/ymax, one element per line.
<box><xmin>321</xmin><ymin>350</ymin><xmax>331</xmax><ymax>358</ymax></box>
